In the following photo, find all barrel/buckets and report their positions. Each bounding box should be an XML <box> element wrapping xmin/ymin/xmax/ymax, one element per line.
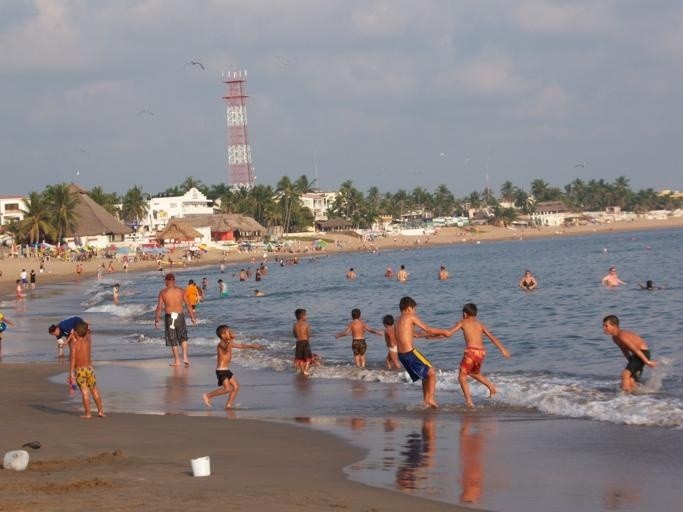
<box><xmin>189</xmin><ymin>454</ymin><xmax>211</xmax><ymax>478</ymax></box>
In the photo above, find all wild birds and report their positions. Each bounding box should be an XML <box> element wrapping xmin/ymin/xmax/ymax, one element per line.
<box><xmin>185</xmin><ymin>60</ymin><xmax>204</xmax><ymax>72</ymax></box>
<box><xmin>136</xmin><ymin>109</ymin><xmax>154</xmax><ymax>117</ymax></box>
<box><xmin>575</xmin><ymin>163</ymin><xmax>584</xmax><ymax>169</ymax></box>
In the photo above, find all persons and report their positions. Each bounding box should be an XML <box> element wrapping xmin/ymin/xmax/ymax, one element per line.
<box><xmin>0</xmin><ymin>239</ymin><xmax>324</xmax><ymax>333</ymax></box>
<box><xmin>201</xmin><ymin>325</ymin><xmax>259</xmax><ymax>410</ymax></box>
<box><xmin>154</xmin><ymin>274</ymin><xmax>197</xmax><ymax>367</ymax></box>
<box><xmin>333</xmin><ymin>300</ymin><xmax>511</xmax><ymax>408</ymax></box>
<box><xmin>439</xmin><ymin>265</ymin><xmax>449</xmax><ymax>279</ymax></box>
<box><xmin>47</xmin><ymin>317</ymin><xmax>83</xmax><ymax>357</ymax></box>
<box><xmin>519</xmin><ymin>270</ymin><xmax>538</xmax><ymax>290</ymax></box>
<box><xmin>385</xmin><ymin>268</ymin><xmax>393</xmax><ymax>277</ymax></box>
<box><xmin>346</xmin><ymin>268</ymin><xmax>356</xmax><ymax>280</ymax></box>
<box><xmin>456</xmin><ymin>412</ymin><xmax>487</xmax><ymax>504</ymax></box>
<box><xmin>601</xmin><ymin>317</ymin><xmax>657</xmax><ymax>395</ymax></box>
<box><xmin>602</xmin><ymin>267</ymin><xmax>627</xmax><ymax>288</ymax></box>
<box><xmin>69</xmin><ymin>321</ymin><xmax>106</xmax><ymax>418</ymax></box>
<box><xmin>395</xmin><ymin>416</ymin><xmax>435</xmax><ymax>496</ymax></box>
<box><xmin>397</xmin><ymin>266</ymin><xmax>406</xmax><ymax>281</ymax></box>
<box><xmin>640</xmin><ymin>280</ymin><xmax>662</xmax><ymax>292</ymax></box>
<box><xmin>293</xmin><ymin>308</ymin><xmax>314</xmax><ymax>377</ymax></box>
